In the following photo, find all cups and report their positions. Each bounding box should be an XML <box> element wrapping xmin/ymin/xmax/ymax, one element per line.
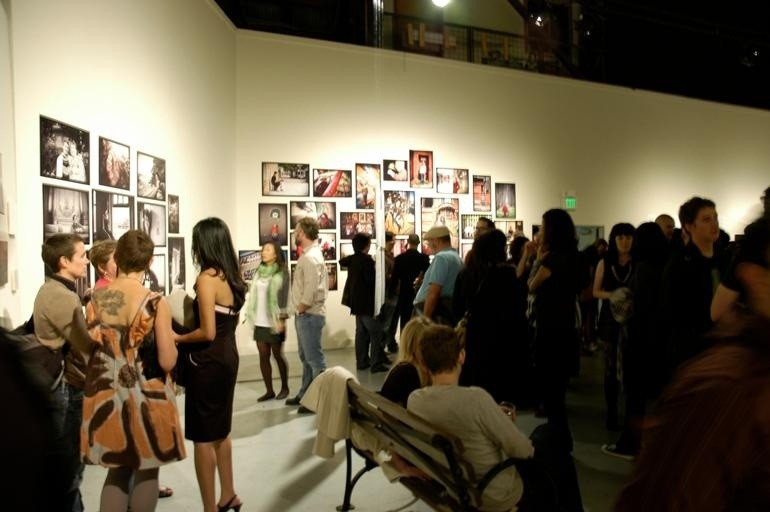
<box><xmin>500</xmin><ymin>401</ymin><xmax>515</xmax><ymax>417</ymax></box>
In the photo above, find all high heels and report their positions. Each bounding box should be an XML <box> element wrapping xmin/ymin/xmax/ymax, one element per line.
<box><xmin>216</xmin><ymin>493</ymin><xmax>242</xmax><ymax>512</ymax></box>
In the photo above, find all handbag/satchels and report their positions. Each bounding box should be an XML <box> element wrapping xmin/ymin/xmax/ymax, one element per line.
<box><xmin>18</xmin><ymin>333</ymin><xmax>66</xmax><ymax>393</ymax></box>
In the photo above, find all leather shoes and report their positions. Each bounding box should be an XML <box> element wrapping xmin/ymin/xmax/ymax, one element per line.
<box><xmin>256</xmin><ymin>390</ymin><xmax>275</xmax><ymax>402</ymax></box>
<box><xmin>276</xmin><ymin>389</ymin><xmax>290</xmax><ymax>400</ymax></box>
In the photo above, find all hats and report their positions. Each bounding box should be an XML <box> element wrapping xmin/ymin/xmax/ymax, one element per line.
<box><xmin>422</xmin><ymin>225</ymin><xmax>450</xmax><ymax>241</ymax></box>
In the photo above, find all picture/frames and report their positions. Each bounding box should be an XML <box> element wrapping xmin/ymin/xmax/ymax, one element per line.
<box><xmin>35</xmin><ymin>111</ymin><xmax>187</xmax><ymax>298</ymax></box>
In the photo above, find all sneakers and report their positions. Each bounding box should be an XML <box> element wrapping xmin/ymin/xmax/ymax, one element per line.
<box><xmin>603</xmin><ymin>412</ymin><xmax>626</xmax><ymax>431</ymax></box>
<box><xmin>354</xmin><ymin>349</ymin><xmax>400</xmax><ymax>374</ymax></box>
<box><xmin>298</xmin><ymin>406</ymin><xmax>316</xmax><ymax>414</ymax></box>
<box><xmin>285</xmin><ymin>396</ymin><xmax>303</xmax><ymax>406</ymax></box>
<box><xmin>599</xmin><ymin>441</ymin><xmax>638</xmax><ymax>461</ymax></box>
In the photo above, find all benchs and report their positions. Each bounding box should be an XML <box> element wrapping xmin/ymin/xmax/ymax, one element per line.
<box><xmin>314</xmin><ymin>367</ymin><xmax>526</xmax><ymax>510</ymax></box>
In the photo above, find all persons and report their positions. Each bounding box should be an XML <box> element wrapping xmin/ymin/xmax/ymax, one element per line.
<box><xmin>106</xmin><ymin>152</ymin><xmax>129</xmax><ymax>190</ymax></box>
<box><xmin>452</xmin><ymin>177</ymin><xmax>460</xmax><ymax>193</ymax></box>
<box><xmin>243</xmin><ymin>240</ymin><xmax>291</xmax><ymax>403</ymax></box>
<box><xmin>138</xmin><ymin>202</ymin><xmax>163</xmax><ymax>245</ymax></box>
<box><xmin>101</xmin><ymin>207</ymin><xmax>117</xmax><ymax>242</ymax></box>
<box><xmin>1</xmin><ymin>325</ymin><xmax>75</xmax><ymax>511</ymax></box>
<box><xmin>170</xmin><ymin>216</ymin><xmax>252</xmax><ymax>511</ymax></box>
<box><xmin>388</xmin><ymin>161</ymin><xmax>407</xmax><ymax>181</ymax></box>
<box><xmin>383</xmin><ymin>160</ymin><xmax>398</xmax><ymax>180</ymax></box>
<box><xmin>270</xmin><ymin>171</ymin><xmax>281</xmax><ymax>191</ymax></box>
<box><xmin>76</xmin><ymin>226</ymin><xmax>189</xmax><ymax>511</ymax></box>
<box><xmin>418</xmin><ymin>155</ymin><xmax>428</xmax><ymax>182</ymax></box>
<box><xmin>67</xmin><ymin>138</ymin><xmax>89</xmax><ymax>183</ymax></box>
<box><xmin>338</xmin><ymin>186</ymin><xmax>769</xmax><ymax>511</ymax></box>
<box><xmin>55</xmin><ymin>139</ymin><xmax>72</xmax><ymax>182</ymax></box>
<box><xmin>85</xmin><ymin>241</ymin><xmax>120</xmax><ymax>291</ymax></box>
<box><xmin>151</xmin><ymin>170</ymin><xmax>165</xmax><ymax>200</ymax></box>
<box><xmin>31</xmin><ymin>233</ymin><xmax>99</xmax><ymax>512</ymax></box>
<box><xmin>284</xmin><ymin>215</ymin><xmax>331</xmax><ymax>417</ymax></box>
<box><xmin>72</xmin><ymin>215</ymin><xmax>85</xmax><ymax>233</ymax></box>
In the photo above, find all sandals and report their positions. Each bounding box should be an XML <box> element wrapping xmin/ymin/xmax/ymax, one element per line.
<box><xmin>158</xmin><ymin>486</ymin><xmax>174</xmax><ymax>498</ymax></box>
